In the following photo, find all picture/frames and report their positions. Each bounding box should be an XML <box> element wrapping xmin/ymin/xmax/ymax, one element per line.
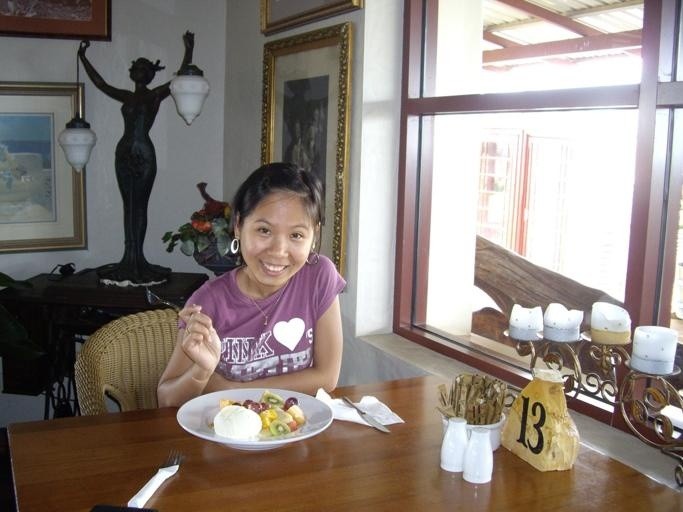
<box><xmin>260</xmin><ymin>21</ymin><xmax>354</xmax><ymax>293</ymax></box>
<box><xmin>258</xmin><ymin>0</ymin><xmax>364</xmax><ymax>37</ymax></box>
<box><xmin>0</xmin><ymin>0</ymin><xmax>113</xmax><ymax>42</ymax></box>
<box><xmin>0</xmin><ymin>80</ymin><xmax>88</xmax><ymax>253</ymax></box>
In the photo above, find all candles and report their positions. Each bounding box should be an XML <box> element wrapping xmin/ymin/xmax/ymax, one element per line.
<box><xmin>543</xmin><ymin>302</ymin><xmax>585</xmax><ymax>342</ymax></box>
<box><xmin>509</xmin><ymin>303</ymin><xmax>543</xmax><ymax>342</ymax></box>
<box><xmin>591</xmin><ymin>301</ymin><xmax>630</xmax><ymax>343</ymax></box>
<box><xmin>629</xmin><ymin>325</ymin><xmax>678</xmax><ymax>362</ymax></box>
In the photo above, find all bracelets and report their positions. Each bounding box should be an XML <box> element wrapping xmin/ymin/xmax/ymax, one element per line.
<box><xmin>191</xmin><ymin>377</ymin><xmax>210</xmax><ymax>383</ymax></box>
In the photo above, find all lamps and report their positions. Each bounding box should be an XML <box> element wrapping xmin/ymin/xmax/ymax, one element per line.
<box><xmin>57</xmin><ymin>30</ymin><xmax>211</xmax><ymax>287</ymax></box>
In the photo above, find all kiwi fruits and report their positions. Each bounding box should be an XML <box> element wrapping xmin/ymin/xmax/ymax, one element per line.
<box><xmin>264</xmin><ymin>394</ymin><xmax>284</xmax><ymax>408</ymax></box>
<box><xmin>269</xmin><ymin>420</ymin><xmax>291</xmax><ymax>436</ymax></box>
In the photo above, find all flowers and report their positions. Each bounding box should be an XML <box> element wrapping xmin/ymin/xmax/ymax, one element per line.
<box><xmin>160</xmin><ymin>209</ymin><xmax>243</xmax><ymax>275</ymax></box>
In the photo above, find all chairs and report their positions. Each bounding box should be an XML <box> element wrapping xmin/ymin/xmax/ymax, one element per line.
<box><xmin>73</xmin><ymin>309</ymin><xmax>178</xmax><ymax>416</ymax></box>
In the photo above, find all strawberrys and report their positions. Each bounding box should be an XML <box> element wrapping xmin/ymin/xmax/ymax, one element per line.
<box><xmin>287</xmin><ymin>420</ymin><xmax>297</xmax><ymax>433</ymax></box>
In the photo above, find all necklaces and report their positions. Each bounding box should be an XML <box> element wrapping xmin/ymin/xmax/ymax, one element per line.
<box><xmin>248</xmin><ymin>281</ymin><xmax>288</xmax><ymax>325</ymax></box>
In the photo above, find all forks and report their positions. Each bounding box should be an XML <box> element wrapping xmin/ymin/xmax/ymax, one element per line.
<box><xmin>143</xmin><ymin>285</ymin><xmax>180</xmax><ymax>312</ymax></box>
<box><xmin>162</xmin><ymin>450</ymin><xmax>181</xmax><ymax>466</ymax></box>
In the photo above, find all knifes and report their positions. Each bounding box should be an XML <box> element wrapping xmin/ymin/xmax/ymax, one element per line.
<box><xmin>342</xmin><ymin>395</ymin><xmax>390</xmax><ymax>433</ymax></box>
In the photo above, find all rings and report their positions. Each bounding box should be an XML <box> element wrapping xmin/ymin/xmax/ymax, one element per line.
<box><xmin>184</xmin><ymin>328</ymin><xmax>190</xmax><ymax>336</ymax></box>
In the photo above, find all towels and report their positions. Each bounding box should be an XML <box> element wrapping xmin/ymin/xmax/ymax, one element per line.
<box><xmin>315</xmin><ymin>387</ymin><xmax>406</xmax><ymax>426</ymax></box>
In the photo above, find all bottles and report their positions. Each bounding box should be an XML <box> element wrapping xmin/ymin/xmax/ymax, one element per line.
<box><xmin>439</xmin><ymin>417</ymin><xmax>469</xmax><ymax>473</ymax></box>
<box><xmin>462</xmin><ymin>426</ymin><xmax>493</xmax><ymax>484</ymax></box>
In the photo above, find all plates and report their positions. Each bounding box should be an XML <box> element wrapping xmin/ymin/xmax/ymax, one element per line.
<box><xmin>175</xmin><ymin>388</ymin><xmax>333</xmax><ymax>450</ymax></box>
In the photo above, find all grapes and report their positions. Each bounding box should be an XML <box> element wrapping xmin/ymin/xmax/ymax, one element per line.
<box><xmin>243</xmin><ymin>399</ymin><xmax>253</xmax><ymax>409</ymax></box>
<box><xmin>284</xmin><ymin>397</ymin><xmax>298</xmax><ymax>410</ymax></box>
<box><xmin>259</xmin><ymin>403</ymin><xmax>272</xmax><ymax>412</ymax></box>
<box><xmin>248</xmin><ymin>402</ymin><xmax>262</xmax><ymax>414</ymax></box>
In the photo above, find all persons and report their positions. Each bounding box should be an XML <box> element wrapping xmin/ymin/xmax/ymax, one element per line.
<box><xmin>156</xmin><ymin>162</ymin><xmax>348</xmax><ymax>409</ymax></box>
<box><xmin>79</xmin><ymin>29</ymin><xmax>195</xmax><ymax>286</ymax></box>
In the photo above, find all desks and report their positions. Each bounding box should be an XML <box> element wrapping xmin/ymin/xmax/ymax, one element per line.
<box><xmin>19</xmin><ymin>263</ymin><xmax>209</xmax><ymax>421</ymax></box>
